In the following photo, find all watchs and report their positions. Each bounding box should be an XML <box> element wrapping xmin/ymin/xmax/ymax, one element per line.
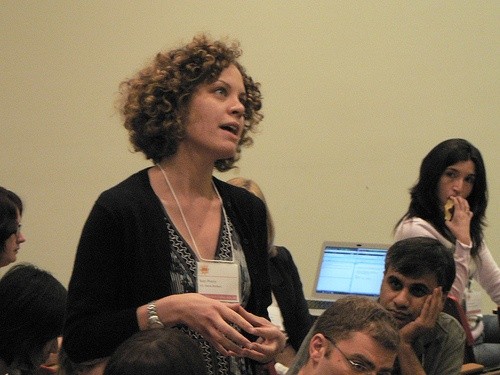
<box><xmin>146</xmin><ymin>300</ymin><xmax>164</xmax><ymax>329</ymax></box>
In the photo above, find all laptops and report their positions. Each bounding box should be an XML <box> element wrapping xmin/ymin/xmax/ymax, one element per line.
<box><xmin>306</xmin><ymin>241</ymin><xmax>390</xmax><ymax>318</ymax></box>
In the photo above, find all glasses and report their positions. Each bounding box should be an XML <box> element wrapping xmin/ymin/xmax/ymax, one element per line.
<box><xmin>312</xmin><ymin>330</ymin><xmax>392</xmax><ymax>375</ymax></box>
<box><xmin>13</xmin><ymin>224</ymin><xmax>22</xmax><ymax>237</ymax></box>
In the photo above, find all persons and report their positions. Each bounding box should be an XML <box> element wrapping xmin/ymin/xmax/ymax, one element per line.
<box><xmin>0</xmin><ymin>185</ymin><xmax>73</xmax><ymax>375</ymax></box>
<box><xmin>297</xmin><ymin>297</ymin><xmax>401</xmax><ymax>375</ymax></box>
<box><xmin>391</xmin><ymin>138</ymin><xmax>500</xmax><ymax>367</ymax></box>
<box><xmin>223</xmin><ymin>178</ymin><xmax>314</xmax><ymax>375</ymax></box>
<box><xmin>65</xmin><ymin>36</ymin><xmax>289</xmax><ymax>375</ymax></box>
<box><xmin>378</xmin><ymin>236</ymin><xmax>465</xmax><ymax>375</ymax></box>
